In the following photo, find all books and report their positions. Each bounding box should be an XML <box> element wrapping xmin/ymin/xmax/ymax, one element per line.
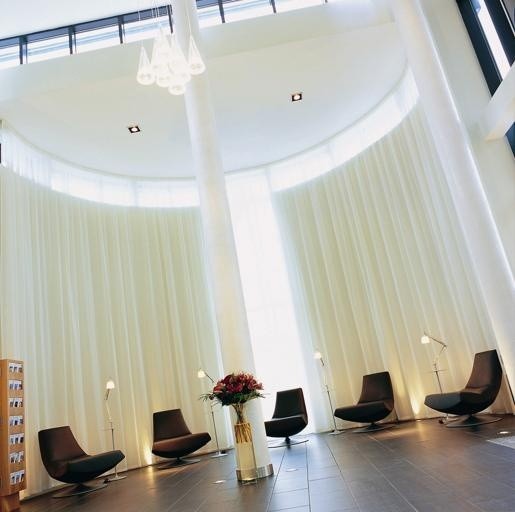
<box><xmin>8</xmin><ymin>363</ymin><xmax>25</xmax><ymax>486</ymax></box>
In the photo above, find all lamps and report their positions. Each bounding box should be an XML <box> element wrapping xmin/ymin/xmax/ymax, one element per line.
<box><xmin>312</xmin><ymin>346</ymin><xmax>346</xmax><ymax>436</ymax></box>
<box><xmin>197</xmin><ymin>367</ymin><xmax>228</xmax><ymax>458</ymax></box>
<box><xmin>137</xmin><ymin>0</ymin><xmax>207</xmax><ymax>96</ymax></box>
<box><xmin>421</xmin><ymin>332</ymin><xmax>457</xmax><ymax>424</ymax></box>
<box><xmin>102</xmin><ymin>376</ymin><xmax>127</xmax><ymax>482</ymax></box>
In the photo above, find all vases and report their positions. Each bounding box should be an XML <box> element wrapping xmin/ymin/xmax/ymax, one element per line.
<box><xmin>232</xmin><ymin>421</ymin><xmax>258</xmax><ymax>482</ymax></box>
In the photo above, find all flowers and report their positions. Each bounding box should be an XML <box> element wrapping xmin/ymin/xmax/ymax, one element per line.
<box><xmin>198</xmin><ymin>371</ymin><xmax>264</xmax><ymax>443</ymax></box>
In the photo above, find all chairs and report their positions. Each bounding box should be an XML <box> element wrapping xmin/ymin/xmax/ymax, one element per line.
<box><xmin>334</xmin><ymin>371</ymin><xmax>398</xmax><ymax>433</ymax></box>
<box><xmin>264</xmin><ymin>388</ymin><xmax>309</xmax><ymax>448</ymax></box>
<box><xmin>38</xmin><ymin>427</ymin><xmax>125</xmax><ymax>499</ymax></box>
<box><xmin>151</xmin><ymin>408</ymin><xmax>212</xmax><ymax>469</ymax></box>
<box><xmin>422</xmin><ymin>349</ymin><xmax>504</xmax><ymax>427</ymax></box>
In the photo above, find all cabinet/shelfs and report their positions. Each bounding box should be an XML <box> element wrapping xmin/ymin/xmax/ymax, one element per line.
<box><xmin>0</xmin><ymin>360</ymin><xmax>27</xmax><ymax>512</ymax></box>
